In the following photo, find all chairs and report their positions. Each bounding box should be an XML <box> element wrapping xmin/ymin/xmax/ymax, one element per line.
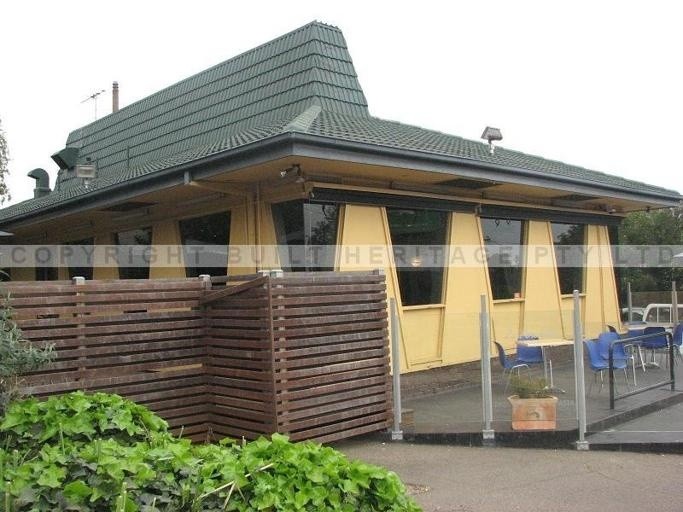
<box><xmin>493</xmin><ymin>321</ymin><xmax>683</xmax><ymax>395</ymax></box>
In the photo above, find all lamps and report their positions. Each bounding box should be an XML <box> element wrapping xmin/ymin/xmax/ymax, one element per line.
<box><xmin>76</xmin><ymin>164</ymin><xmax>96</xmax><ymax>190</ymax></box>
<box><xmin>280</xmin><ymin>163</ymin><xmax>306</xmax><ymax>184</ymax></box>
<box><xmin>481</xmin><ymin>127</ymin><xmax>502</xmax><ymax>156</ymax></box>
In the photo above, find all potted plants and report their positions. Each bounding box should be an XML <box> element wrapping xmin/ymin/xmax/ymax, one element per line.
<box><xmin>505</xmin><ymin>372</ymin><xmax>559</xmax><ymax>432</ymax></box>
